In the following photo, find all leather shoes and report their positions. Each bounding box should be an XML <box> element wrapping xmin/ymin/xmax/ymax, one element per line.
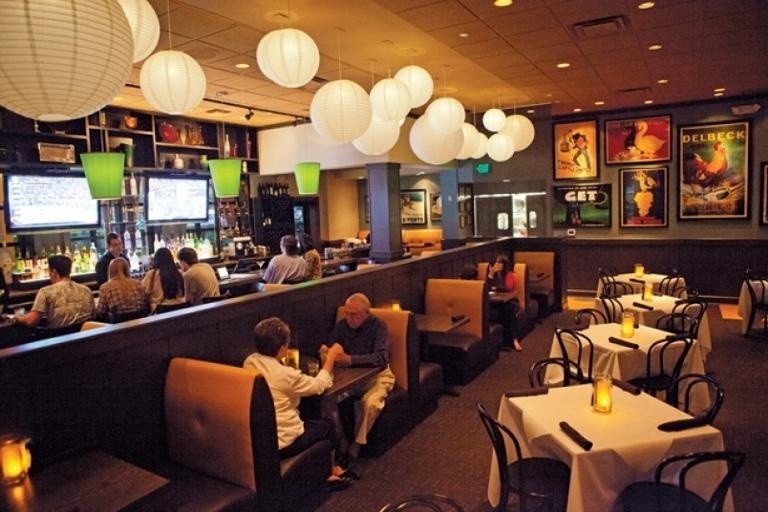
<box><xmin>324</xmin><ymin>450</ymin><xmax>364</xmax><ymax>492</ymax></box>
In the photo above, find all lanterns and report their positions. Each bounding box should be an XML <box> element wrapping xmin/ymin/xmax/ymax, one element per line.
<box><xmin>307</xmin><ymin>64</ymin><xmax>537</xmax><ymax>165</ymax></box>
<box><xmin>1</xmin><ymin>0</ymin><xmax>136</xmax><ymax>125</ymax></box>
<box><xmin>140</xmin><ymin>49</ymin><xmax>209</xmax><ymax>115</ymax></box>
<box><xmin>255</xmin><ymin>28</ymin><xmax>320</xmax><ymax>90</ymax></box>
<box><xmin>119</xmin><ymin>1</ymin><xmax>160</xmax><ymax>64</ymax></box>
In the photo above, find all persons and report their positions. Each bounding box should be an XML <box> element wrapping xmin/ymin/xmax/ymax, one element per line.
<box><xmin>15</xmin><ymin>256</ymin><xmax>96</xmax><ymax>328</ymax></box>
<box><xmin>460</xmin><ymin>255</ymin><xmax>523</xmax><ymax>352</ymax></box>
<box><xmin>300</xmin><ymin>233</ymin><xmax>322</xmax><ymax>281</ymax></box>
<box><xmin>93</xmin><ymin>257</ymin><xmax>145</xmax><ymax>324</ymax></box>
<box><xmin>262</xmin><ymin>235</ymin><xmax>307</xmax><ymax>284</ymax></box>
<box><xmin>140</xmin><ymin>248</ymin><xmax>186</xmax><ymax>312</ymax></box>
<box><xmin>319</xmin><ymin>294</ymin><xmax>396</xmax><ymax>475</ymax></box>
<box><xmin>177</xmin><ymin>247</ymin><xmax>220</xmax><ymax>305</ymax></box>
<box><xmin>95</xmin><ymin>233</ymin><xmax>130</xmax><ymax>285</ymax></box>
<box><xmin>243</xmin><ymin>317</ymin><xmax>351</xmax><ymax>493</ymax></box>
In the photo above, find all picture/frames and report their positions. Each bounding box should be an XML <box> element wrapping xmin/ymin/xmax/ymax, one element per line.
<box><xmin>365</xmin><ymin>195</ymin><xmax>370</xmax><ymax>224</ymax></box>
<box><xmin>430</xmin><ymin>193</ymin><xmax>443</xmax><ymax>222</ymax></box>
<box><xmin>400</xmin><ymin>189</ymin><xmax>427</xmax><ymax>226</ymax></box>
<box><xmin>548</xmin><ymin>110</ymin><xmax>768</xmax><ymax>229</ymax></box>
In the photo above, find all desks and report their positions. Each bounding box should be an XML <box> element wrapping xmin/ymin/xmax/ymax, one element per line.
<box><xmin>402</xmin><ymin>242</ymin><xmax>433</xmax><ymax>254</ymax></box>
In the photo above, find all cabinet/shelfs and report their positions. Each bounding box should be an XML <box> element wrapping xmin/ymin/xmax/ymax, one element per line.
<box><xmin>251</xmin><ymin>194</ymin><xmax>322</xmax><ymax>253</ymax></box>
<box><xmin>1</xmin><ymin>102</ymin><xmax>260</xmax><ymax>307</ymax></box>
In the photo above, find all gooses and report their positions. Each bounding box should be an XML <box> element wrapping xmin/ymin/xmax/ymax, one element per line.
<box><xmin>634</xmin><ymin>121</ymin><xmax>665</xmax><ymax>154</ymax></box>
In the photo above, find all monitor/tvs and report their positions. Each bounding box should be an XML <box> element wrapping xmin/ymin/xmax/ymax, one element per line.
<box><xmin>2</xmin><ymin>170</ymin><xmax>101</xmax><ymax>233</ymax></box>
<box><xmin>145</xmin><ymin>176</ymin><xmax>210</xmax><ymax>224</ymax></box>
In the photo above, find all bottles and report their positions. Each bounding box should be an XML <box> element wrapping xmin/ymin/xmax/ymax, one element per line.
<box><xmin>559</xmin><ymin>133</ymin><xmax>569</xmax><ymax>152</ymax></box>
<box><xmin>197</xmin><ymin>155</ymin><xmax>210</xmax><ymax>172</ymax></box>
<box><xmin>242</xmin><ymin>131</ymin><xmax>252</xmax><ymax>159</ymax></box>
<box><xmin>171</xmin><ymin>154</ymin><xmax>187</xmax><ymax>171</ymax></box>
<box><xmin>100</xmin><ymin>196</ymin><xmax>147</xmax><ymax>275</ymax></box>
<box><xmin>4</xmin><ymin>238</ymin><xmax>99</xmax><ymax>278</ymax></box>
<box><xmin>222</xmin><ymin>133</ymin><xmax>232</xmax><ymax>158</ymax></box>
<box><xmin>151</xmin><ymin>226</ymin><xmax>214</xmax><ymax>262</ymax></box>
<box><xmin>256</xmin><ymin>180</ymin><xmax>292</xmax><ymax>199</ymax></box>
<box><xmin>127</xmin><ymin>169</ymin><xmax>139</xmax><ymax>197</ymax></box>
<box><xmin>216</xmin><ymin>198</ymin><xmax>252</xmax><ymax>257</ymax></box>
<box><xmin>231</xmin><ymin>141</ymin><xmax>239</xmax><ymax>157</ymax></box>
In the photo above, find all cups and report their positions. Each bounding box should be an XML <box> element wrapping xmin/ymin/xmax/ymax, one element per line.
<box><xmin>592</xmin><ymin>373</ymin><xmax>613</xmax><ymax>413</ymax></box>
<box><xmin>634</xmin><ymin>263</ymin><xmax>645</xmax><ymax>278</ymax></box>
<box><xmin>391</xmin><ymin>303</ymin><xmax>400</xmax><ymax>311</ymax></box>
<box><xmin>620</xmin><ymin>312</ymin><xmax>635</xmax><ymax>338</ymax></box>
<box><xmin>307</xmin><ymin>359</ymin><xmax>320</xmax><ymax>374</ymax></box>
<box><xmin>491</xmin><ymin>286</ymin><xmax>497</xmax><ymax>292</ymax></box>
<box><xmin>644</xmin><ymin>283</ymin><xmax>654</xmax><ymax>302</ymax></box>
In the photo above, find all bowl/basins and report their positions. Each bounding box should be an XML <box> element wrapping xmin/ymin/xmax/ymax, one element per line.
<box><xmin>488</xmin><ymin>292</ymin><xmax>496</xmax><ymax>296</ymax></box>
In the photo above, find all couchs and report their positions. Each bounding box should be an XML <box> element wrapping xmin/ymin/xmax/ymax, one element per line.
<box><xmin>359</xmin><ymin>227</ymin><xmax>443</xmax><ymax>256</ymax></box>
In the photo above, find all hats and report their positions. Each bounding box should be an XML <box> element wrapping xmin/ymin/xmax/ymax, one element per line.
<box><xmin>281</xmin><ymin>235</ymin><xmax>297</xmax><ymax>246</ymax></box>
<box><xmin>296</xmin><ymin>232</ymin><xmax>312</xmax><ymax>247</ymax></box>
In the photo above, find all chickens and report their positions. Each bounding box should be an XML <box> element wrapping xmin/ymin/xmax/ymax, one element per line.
<box><xmin>693</xmin><ymin>141</ymin><xmax>728</xmax><ymax>186</ymax></box>
